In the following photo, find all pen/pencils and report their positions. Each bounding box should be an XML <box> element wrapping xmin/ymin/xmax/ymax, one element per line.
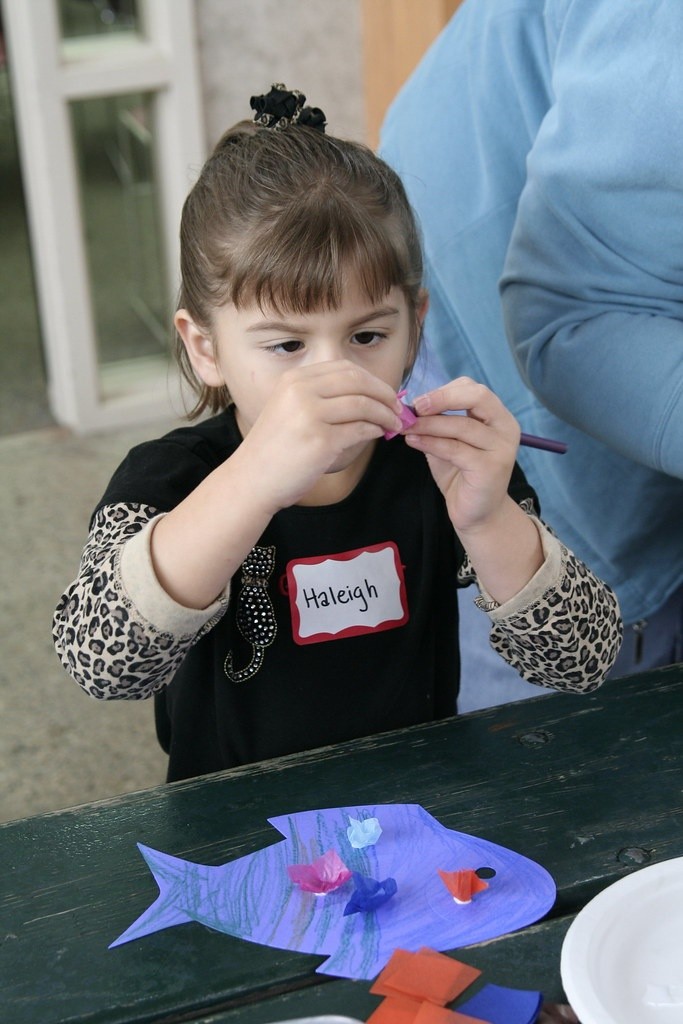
<box><xmin>404</xmin><ymin>405</ymin><xmax>568</xmax><ymax>456</ymax></box>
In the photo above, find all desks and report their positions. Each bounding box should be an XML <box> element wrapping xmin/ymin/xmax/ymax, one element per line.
<box><xmin>0</xmin><ymin>661</ymin><xmax>683</xmax><ymax>1024</ymax></box>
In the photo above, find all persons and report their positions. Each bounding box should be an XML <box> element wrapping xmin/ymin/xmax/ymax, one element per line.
<box><xmin>379</xmin><ymin>0</ymin><xmax>683</xmax><ymax>714</ymax></box>
<box><xmin>52</xmin><ymin>82</ymin><xmax>625</xmax><ymax>783</ymax></box>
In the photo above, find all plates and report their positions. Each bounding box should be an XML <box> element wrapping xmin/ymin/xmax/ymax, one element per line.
<box><xmin>561</xmin><ymin>858</ymin><xmax>682</xmax><ymax>1024</ymax></box>
